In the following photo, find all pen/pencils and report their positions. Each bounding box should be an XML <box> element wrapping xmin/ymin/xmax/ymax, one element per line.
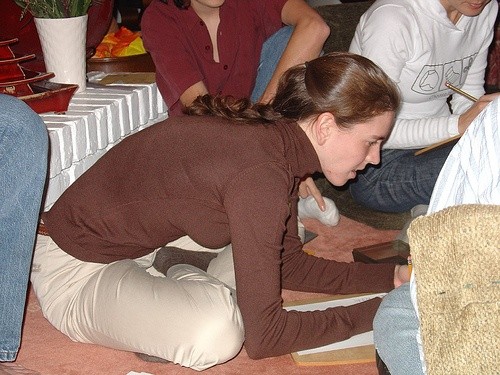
<box><xmin>407</xmin><ymin>256</ymin><xmax>412</xmax><ymax>281</ymax></box>
<box><xmin>415</xmin><ymin>134</ymin><xmax>462</xmax><ymax>155</ymax></box>
<box><xmin>445</xmin><ymin>82</ymin><xmax>477</xmax><ymax>102</ymax></box>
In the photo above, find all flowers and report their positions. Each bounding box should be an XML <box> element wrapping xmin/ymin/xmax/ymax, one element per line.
<box><xmin>14</xmin><ymin>0</ymin><xmax>105</xmax><ymax>23</ymax></box>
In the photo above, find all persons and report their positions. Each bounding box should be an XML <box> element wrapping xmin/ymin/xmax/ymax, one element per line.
<box><xmin>142</xmin><ymin>0</ymin><xmax>500</xmax><ymax>213</ymax></box>
<box><xmin>372</xmin><ymin>95</ymin><xmax>500</xmax><ymax>375</ymax></box>
<box><xmin>29</xmin><ymin>53</ymin><xmax>413</xmax><ymax>372</ymax></box>
<box><xmin>0</xmin><ymin>94</ymin><xmax>49</xmax><ymax>361</ymax></box>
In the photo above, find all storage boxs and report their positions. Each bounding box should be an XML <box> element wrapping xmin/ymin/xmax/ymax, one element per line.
<box><xmin>351</xmin><ymin>240</ymin><xmax>410</xmax><ymax>265</ymax></box>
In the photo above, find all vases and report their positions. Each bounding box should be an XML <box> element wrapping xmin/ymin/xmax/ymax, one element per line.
<box><xmin>33</xmin><ymin>14</ymin><xmax>88</xmax><ymax>92</ymax></box>
